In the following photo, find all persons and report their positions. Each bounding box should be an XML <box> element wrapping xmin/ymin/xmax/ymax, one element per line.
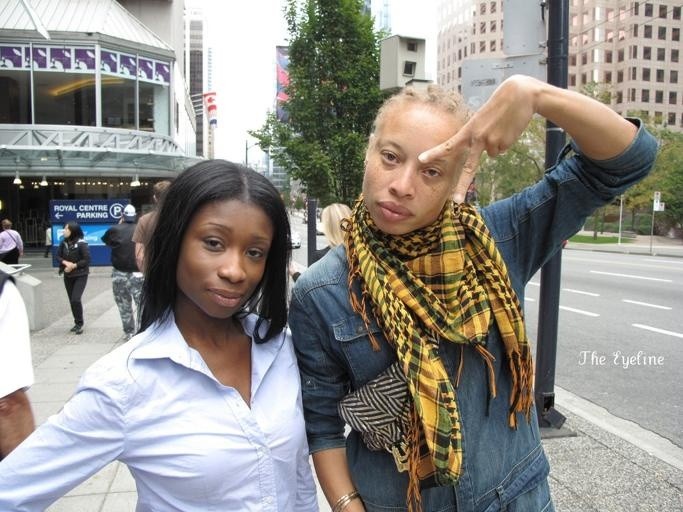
<box><xmin>0</xmin><ymin>159</ymin><xmax>319</xmax><ymax>512</ymax></box>
<box><xmin>289</xmin><ymin>73</ymin><xmax>658</xmax><ymax>512</ymax></box>
<box><xmin>289</xmin><ymin>203</ymin><xmax>352</xmax><ymax>283</ymax></box>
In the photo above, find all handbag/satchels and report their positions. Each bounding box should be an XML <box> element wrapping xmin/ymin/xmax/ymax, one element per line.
<box><xmin>338</xmin><ymin>360</ymin><xmax>421</xmax><ymax>451</ymax></box>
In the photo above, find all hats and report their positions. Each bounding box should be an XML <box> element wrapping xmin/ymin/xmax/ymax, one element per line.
<box><xmin>122</xmin><ymin>204</ymin><xmax>137</xmax><ymax>222</ymax></box>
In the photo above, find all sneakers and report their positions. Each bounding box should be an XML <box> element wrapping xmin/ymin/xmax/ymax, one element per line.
<box><xmin>123</xmin><ymin>334</ymin><xmax>133</xmax><ymax>340</ymax></box>
<box><xmin>70</xmin><ymin>325</ymin><xmax>82</xmax><ymax>334</ymax></box>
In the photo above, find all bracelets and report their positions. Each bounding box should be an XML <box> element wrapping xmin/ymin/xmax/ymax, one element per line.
<box><xmin>332</xmin><ymin>490</ymin><xmax>360</xmax><ymax>512</ymax></box>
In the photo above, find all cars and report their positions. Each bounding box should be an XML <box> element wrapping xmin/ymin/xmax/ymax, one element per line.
<box><xmin>291</xmin><ymin>228</ymin><xmax>301</xmax><ymax>249</ymax></box>
